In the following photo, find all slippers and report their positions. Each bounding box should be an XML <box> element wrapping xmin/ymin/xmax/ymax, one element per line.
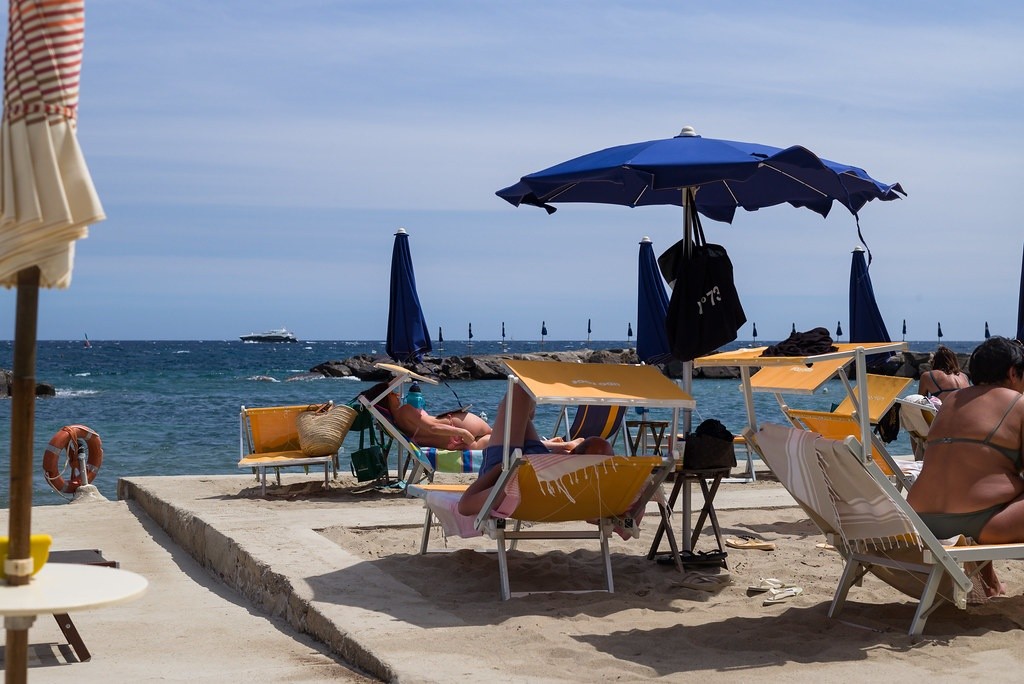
<box><xmin>671</xmin><ymin>571</ymin><xmax>730</xmax><ymax>591</ymax></box>
<box><xmin>657</xmin><ymin>550</ymin><xmax>705</xmax><ymax>563</ymax></box>
<box><xmin>748</xmin><ymin>577</ymin><xmax>796</xmax><ymax>591</ymax></box>
<box><xmin>763</xmin><ymin>586</ymin><xmax>803</xmax><ymax>605</ymax></box>
<box><xmin>726</xmin><ymin>536</ymin><xmax>775</xmax><ymax>549</ymax></box>
<box><xmin>693</xmin><ymin>549</ymin><xmax>727</xmax><ymax>561</ymax></box>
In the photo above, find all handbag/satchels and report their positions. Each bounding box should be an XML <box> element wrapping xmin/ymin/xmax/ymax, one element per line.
<box><xmin>296</xmin><ymin>402</ymin><xmax>358</xmax><ymax>456</ymax></box>
<box><xmin>350</xmin><ymin>427</ymin><xmax>388</xmax><ymax>481</ymax></box>
<box><xmin>348</xmin><ymin>394</ymin><xmax>374</xmax><ymax>431</ymax></box>
<box><xmin>656</xmin><ymin>185</ymin><xmax>747</xmax><ymax>359</ymax></box>
<box><xmin>683</xmin><ymin>431</ymin><xmax>737</xmax><ymax>468</ymax></box>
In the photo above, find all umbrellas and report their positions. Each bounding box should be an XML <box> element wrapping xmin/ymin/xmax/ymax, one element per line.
<box><xmin>385</xmin><ymin>227</ymin><xmax>432</xmax><ymax>478</ymax></box>
<box><xmin>637</xmin><ymin>236</ymin><xmax>674</xmax><ymax>457</ymax></box>
<box><xmin>439</xmin><ymin>319</ymin><xmax>991</xmax><ymax>342</ymax></box>
<box><xmin>848</xmin><ymin>246</ymin><xmax>901</xmax><ymax>376</ymax></box>
<box><xmin>0</xmin><ymin>0</ymin><xmax>107</xmax><ymax>588</ymax></box>
<box><xmin>494</xmin><ymin>126</ymin><xmax>909</xmax><ymax>554</ymax></box>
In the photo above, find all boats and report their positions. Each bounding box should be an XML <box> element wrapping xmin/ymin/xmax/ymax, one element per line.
<box><xmin>239</xmin><ymin>328</ymin><xmax>299</xmax><ymax>343</ymax></box>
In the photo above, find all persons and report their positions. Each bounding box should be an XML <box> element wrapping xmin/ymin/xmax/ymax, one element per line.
<box><xmin>458</xmin><ymin>381</ymin><xmax>613</xmax><ymax>516</ymax></box>
<box><xmin>370</xmin><ymin>383</ymin><xmax>585</xmax><ymax>451</ymax></box>
<box><xmin>907</xmin><ymin>335</ymin><xmax>1024</xmax><ymax>599</ymax></box>
<box><xmin>917</xmin><ymin>348</ymin><xmax>970</xmax><ymax>401</ymax></box>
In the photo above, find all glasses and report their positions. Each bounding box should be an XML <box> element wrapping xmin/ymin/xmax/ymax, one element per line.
<box><xmin>1013</xmin><ymin>339</ymin><xmax>1023</xmax><ymax>348</ymax></box>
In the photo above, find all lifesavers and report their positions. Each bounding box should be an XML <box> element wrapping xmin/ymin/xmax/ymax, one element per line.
<box><xmin>43</xmin><ymin>423</ymin><xmax>104</xmax><ymax>494</ymax></box>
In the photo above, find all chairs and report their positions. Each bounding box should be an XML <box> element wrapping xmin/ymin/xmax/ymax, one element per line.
<box><xmin>356</xmin><ymin>361</ymin><xmax>483</xmax><ymax>500</ymax></box>
<box><xmin>693</xmin><ymin>341</ymin><xmax>1024</xmax><ymax>635</ymax></box>
<box><xmin>46</xmin><ymin>548</ymin><xmax>120</xmax><ymax>662</ymax></box>
<box><xmin>237</xmin><ymin>398</ymin><xmax>344</xmax><ymax>499</ymax></box>
<box><xmin>738</xmin><ymin>356</ymin><xmax>923</xmax><ymax>493</ymax></box>
<box><xmin>832</xmin><ymin>374</ymin><xmax>939</xmax><ymax>451</ymax></box>
<box><xmin>406</xmin><ymin>358</ymin><xmax>696</xmax><ymax>602</ymax></box>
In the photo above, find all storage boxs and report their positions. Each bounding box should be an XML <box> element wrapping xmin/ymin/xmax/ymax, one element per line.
<box><xmin>676</xmin><ymin>440</ymin><xmax>686</xmax><ymax>465</ymax></box>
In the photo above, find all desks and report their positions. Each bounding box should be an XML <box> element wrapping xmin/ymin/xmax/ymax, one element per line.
<box><xmin>646</xmin><ymin>464</ymin><xmax>733</xmax><ymax>574</ymax></box>
<box><xmin>625</xmin><ymin>420</ymin><xmax>671</xmax><ymax>456</ymax></box>
<box><xmin>0</xmin><ymin>562</ymin><xmax>148</xmax><ymax>684</ymax></box>
